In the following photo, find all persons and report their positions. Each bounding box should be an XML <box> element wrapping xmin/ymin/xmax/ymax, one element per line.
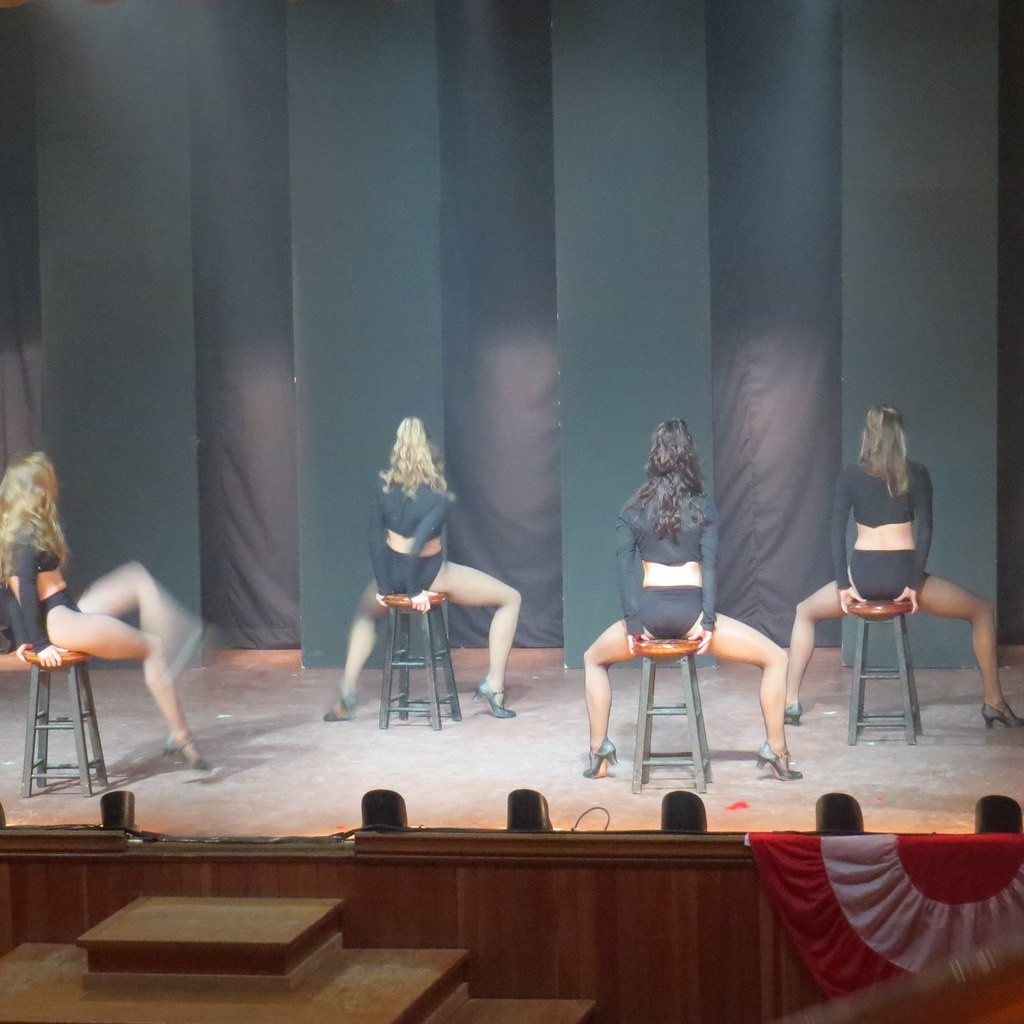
<box><xmin>784</xmin><ymin>403</ymin><xmax>1024</xmax><ymax>729</ymax></box>
<box><xmin>583</xmin><ymin>417</ymin><xmax>802</xmax><ymax>780</ymax></box>
<box><xmin>322</xmin><ymin>416</ymin><xmax>521</xmax><ymax>721</ymax></box>
<box><xmin>0</xmin><ymin>452</ymin><xmax>209</xmax><ymax>772</ymax></box>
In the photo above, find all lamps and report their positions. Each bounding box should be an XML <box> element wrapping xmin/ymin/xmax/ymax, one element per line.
<box><xmin>99</xmin><ymin>791</ymin><xmax>135</xmax><ymax>831</ymax></box>
<box><xmin>661</xmin><ymin>791</ymin><xmax>709</xmax><ymax>833</ymax></box>
<box><xmin>974</xmin><ymin>795</ymin><xmax>1021</xmax><ymax>834</ymax></box>
<box><xmin>362</xmin><ymin>789</ymin><xmax>409</xmax><ymax>831</ymax></box>
<box><xmin>815</xmin><ymin>793</ymin><xmax>865</xmax><ymax>833</ymax></box>
<box><xmin>507</xmin><ymin>789</ymin><xmax>553</xmax><ymax>831</ymax></box>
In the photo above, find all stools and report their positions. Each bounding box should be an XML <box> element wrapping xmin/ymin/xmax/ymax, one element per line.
<box><xmin>21</xmin><ymin>648</ymin><xmax>107</xmax><ymax>798</ymax></box>
<box><xmin>632</xmin><ymin>640</ymin><xmax>714</xmax><ymax>795</ymax></box>
<box><xmin>384</xmin><ymin>593</ymin><xmax>462</xmax><ymax>731</ymax></box>
<box><xmin>848</xmin><ymin>601</ymin><xmax>924</xmax><ymax>746</ymax></box>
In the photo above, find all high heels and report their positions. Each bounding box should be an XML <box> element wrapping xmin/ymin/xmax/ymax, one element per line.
<box><xmin>980</xmin><ymin>701</ymin><xmax>1024</xmax><ymax>730</ymax></box>
<box><xmin>325</xmin><ymin>694</ymin><xmax>357</xmax><ymax>723</ymax></box>
<box><xmin>473</xmin><ymin>681</ymin><xmax>517</xmax><ymax>719</ymax></box>
<box><xmin>783</xmin><ymin>701</ymin><xmax>803</xmax><ymax>727</ymax></box>
<box><xmin>163</xmin><ymin>734</ymin><xmax>212</xmax><ymax>772</ymax></box>
<box><xmin>756</xmin><ymin>742</ymin><xmax>802</xmax><ymax>780</ymax></box>
<box><xmin>584</xmin><ymin>738</ymin><xmax>618</xmax><ymax>779</ymax></box>
